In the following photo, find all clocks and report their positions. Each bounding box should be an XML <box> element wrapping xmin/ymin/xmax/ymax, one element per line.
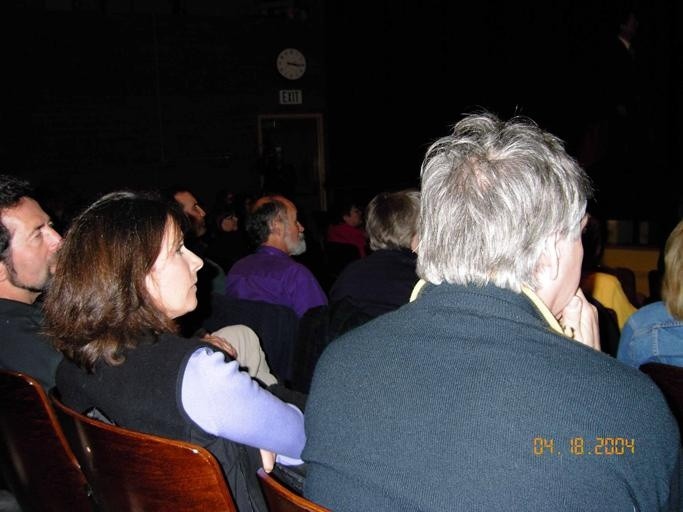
<box><xmin>274</xmin><ymin>47</ymin><xmax>307</xmax><ymax>81</ymax></box>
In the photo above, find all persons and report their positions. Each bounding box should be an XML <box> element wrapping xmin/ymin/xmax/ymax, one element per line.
<box><xmin>301</xmin><ymin>115</ymin><xmax>682</xmax><ymax>511</ymax></box>
<box><xmin>616</xmin><ymin>221</ymin><xmax>682</xmax><ymax>370</ymax></box>
<box><xmin>578</xmin><ymin>224</ymin><xmax>638</xmax><ymax>334</ymax></box>
<box><xmin>327</xmin><ymin>189</ymin><xmax>425</xmax><ymax>318</ymax></box>
<box><xmin>330</xmin><ymin>203</ymin><xmax>372</xmax><ymax>259</ymax></box>
<box><xmin>1</xmin><ymin>177</ymin><xmax>328</xmax><ymax>512</ymax></box>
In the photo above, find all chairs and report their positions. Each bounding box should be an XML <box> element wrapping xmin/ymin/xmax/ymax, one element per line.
<box><xmin>1</xmin><ymin>367</ymin><xmax>94</xmax><ymax>511</ymax></box>
<box><xmin>256</xmin><ymin>466</ymin><xmax>334</xmax><ymax>511</ymax></box>
<box><xmin>49</xmin><ymin>388</ymin><xmax>236</xmax><ymax>511</ymax></box>
